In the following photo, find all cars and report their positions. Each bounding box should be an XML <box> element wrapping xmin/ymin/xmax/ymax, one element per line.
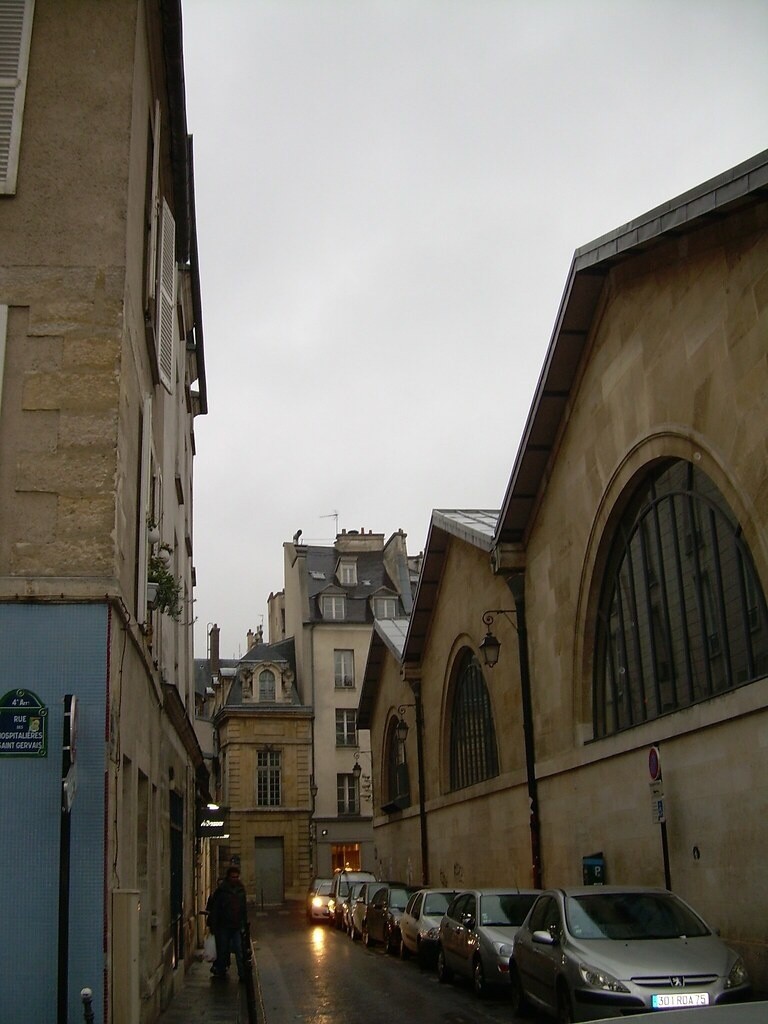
<box><xmin>308</xmin><ymin>875</ymin><xmax>333</xmax><ymax>921</ymax></box>
<box><xmin>343</xmin><ymin>881</ymin><xmax>423</xmax><ymax>955</ymax></box>
<box><xmin>508</xmin><ymin>885</ymin><xmax>753</xmax><ymax>1024</ymax></box>
<box><xmin>401</xmin><ymin>888</ymin><xmax>461</xmax><ymax>969</ymax></box>
<box><xmin>435</xmin><ymin>886</ymin><xmax>547</xmax><ymax>1002</ymax></box>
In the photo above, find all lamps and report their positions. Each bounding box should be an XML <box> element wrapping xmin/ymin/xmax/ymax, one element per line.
<box><xmin>309</xmin><ymin>773</ymin><xmax>318</xmax><ymax>797</ymax></box>
<box><xmin>478</xmin><ymin>609</ymin><xmax>518</xmax><ymax>669</ymax></box>
<box><xmin>395</xmin><ymin>704</ymin><xmax>416</xmax><ymax>744</ymax></box>
<box><xmin>352</xmin><ymin>750</ymin><xmax>370</xmax><ymax>780</ymax></box>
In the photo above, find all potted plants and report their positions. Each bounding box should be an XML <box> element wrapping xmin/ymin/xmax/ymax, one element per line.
<box><xmin>147</xmin><ymin>555</ymin><xmax>199</xmax><ymax>626</ymax></box>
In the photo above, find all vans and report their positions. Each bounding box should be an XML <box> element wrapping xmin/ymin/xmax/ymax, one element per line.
<box><xmin>329</xmin><ymin>871</ymin><xmax>376</xmax><ymax>928</ymax></box>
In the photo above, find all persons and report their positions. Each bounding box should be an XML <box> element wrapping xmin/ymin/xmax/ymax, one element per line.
<box><xmin>203</xmin><ymin>865</ymin><xmax>251</xmax><ymax>989</ymax></box>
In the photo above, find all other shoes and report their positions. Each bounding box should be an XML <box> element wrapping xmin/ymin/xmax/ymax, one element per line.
<box><xmin>239</xmin><ymin>977</ymin><xmax>249</xmax><ymax>982</ymax></box>
<box><xmin>218</xmin><ymin>971</ymin><xmax>227</xmax><ymax>979</ymax></box>
